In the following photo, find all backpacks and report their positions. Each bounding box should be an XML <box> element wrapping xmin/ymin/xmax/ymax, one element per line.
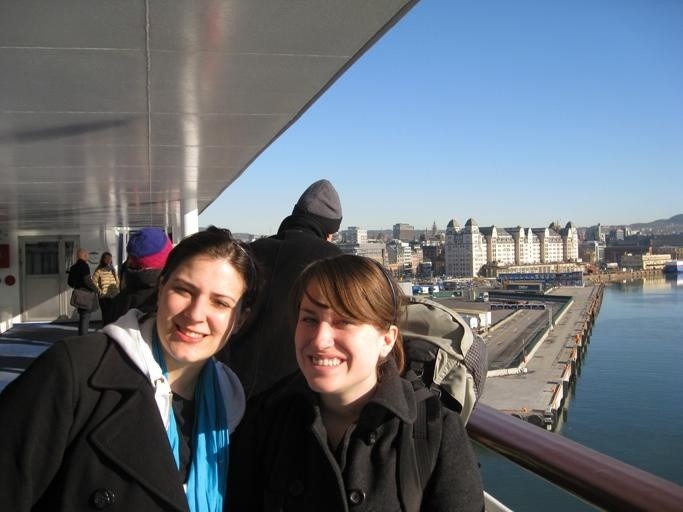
<box><xmin>397</xmin><ymin>295</ymin><xmax>490</xmax><ymax>429</ymax></box>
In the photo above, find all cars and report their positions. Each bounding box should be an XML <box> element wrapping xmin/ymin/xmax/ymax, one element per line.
<box><xmin>437</xmin><ymin>280</ymin><xmax>474</xmax><ymax>291</ymax></box>
<box><xmin>412</xmin><ymin>286</ymin><xmax>423</xmax><ymax>294</ymax></box>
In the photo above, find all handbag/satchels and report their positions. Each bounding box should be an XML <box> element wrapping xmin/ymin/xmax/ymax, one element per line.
<box><xmin>70</xmin><ymin>288</ymin><xmax>99</xmax><ymax>314</ymax></box>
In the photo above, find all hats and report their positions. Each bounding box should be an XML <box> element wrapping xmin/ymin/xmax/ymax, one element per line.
<box><xmin>125</xmin><ymin>227</ymin><xmax>172</xmax><ymax>270</ymax></box>
<box><xmin>292</xmin><ymin>178</ymin><xmax>343</xmax><ymax>233</ymax></box>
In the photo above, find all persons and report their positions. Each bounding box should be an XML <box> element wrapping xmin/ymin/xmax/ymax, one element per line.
<box><xmin>108</xmin><ymin>227</ymin><xmax>173</xmax><ymax>324</ymax></box>
<box><xmin>91</xmin><ymin>251</ymin><xmax>120</xmax><ymax>327</ymax></box>
<box><xmin>1</xmin><ymin>225</ymin><xmax>260</xmax><ymax>510</ymax></box>
<box><xmin>210</xmin><ymin>178</ymin><xmax>348</xmax><ymax>405</ymax></box>
<box><xmin>66</xmin><ymin>247</ymin><xmax>99</xmax><ymax>337</ymax></box>
<box><xmin>221</xmin><ymin>252</ymin><xmax>484</xmax><ymax>511</ymax></box>
